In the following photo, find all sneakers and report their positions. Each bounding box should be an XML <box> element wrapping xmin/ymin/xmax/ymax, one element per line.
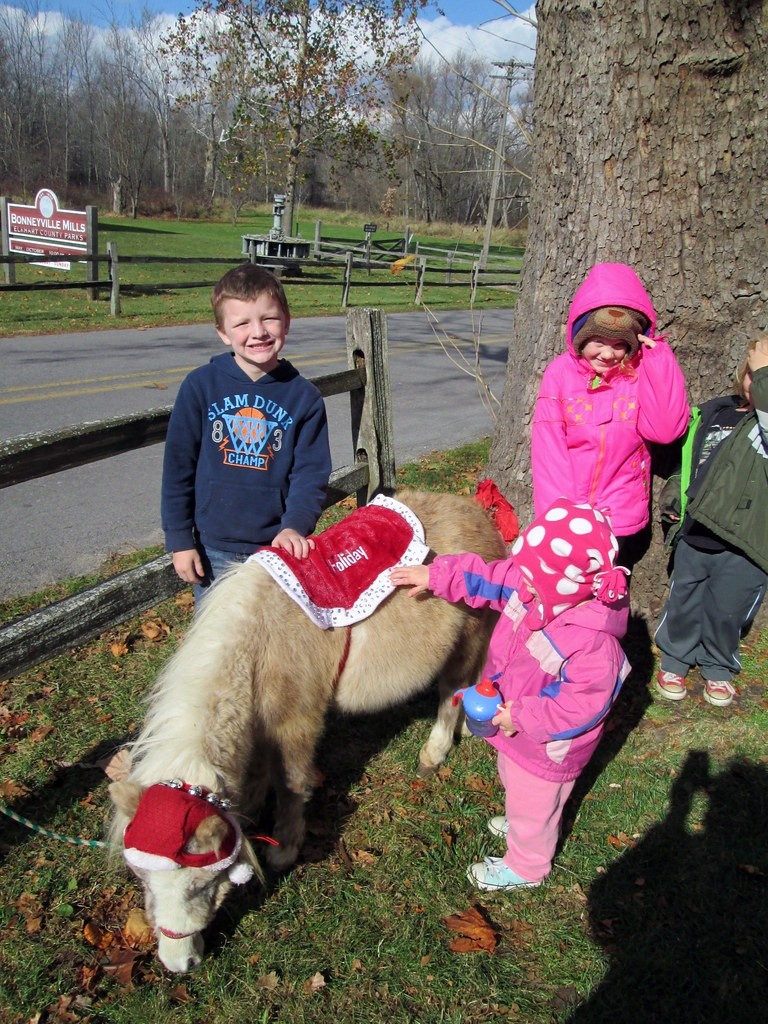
<box><xmin>487</xmin><ymin>816</ymin><xmax>509</xmax><ymax>838</ymax></box>
<box><xmin>658</xmin><ymin>664</ymin><xmax>687</xmax><ymax>700</ymax></box>
<box><xmin>466</xmin><ymin>856</ymin><xmax>541</xmax><ymax>891</ymax></box>
<box><xmin>703</xmin><ymin>679</ymin><xmax>736</xmax><ymax>707</ymax></box>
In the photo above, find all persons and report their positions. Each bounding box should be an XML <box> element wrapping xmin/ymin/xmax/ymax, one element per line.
<box><xmin>531</xmin><ymin>264</ymin><xmax>690</xmax><ymax>547</ymax></box>
<box><xmin>161</xmin><ymin>263</ymin><xmax>332</xmax><ymax>608</ymax></box>
<box><xmin>653</xmin><ymin>333</ymin><xmax>767</xmax><ymax>705</ymax></box>
<box><xmin>388</xmin><ymin>496</ymin><xmax>632</xmax><ymax>891</ymax></box>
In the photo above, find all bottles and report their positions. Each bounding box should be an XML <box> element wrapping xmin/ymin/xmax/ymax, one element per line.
<box><xmin>452</xmin><ymin>678</ymin><xmax>506</xmax><ymax>738</ymax></box>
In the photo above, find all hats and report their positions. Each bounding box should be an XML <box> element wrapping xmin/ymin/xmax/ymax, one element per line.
<box><xmin>570</xmin><ymin>307</ymin><xmax>652</xmax><ymax>367</ymax></box>
<box><xmin>509</xmin><ymin>496</ymin><xmax>629</xmax><ymax>630</ymax></box>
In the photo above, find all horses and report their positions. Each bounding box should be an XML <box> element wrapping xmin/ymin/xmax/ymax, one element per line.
<box><xmin>105</xmin><ymin>487</ymin><xmax>504</xmax><ymax>976</ymax></box>
